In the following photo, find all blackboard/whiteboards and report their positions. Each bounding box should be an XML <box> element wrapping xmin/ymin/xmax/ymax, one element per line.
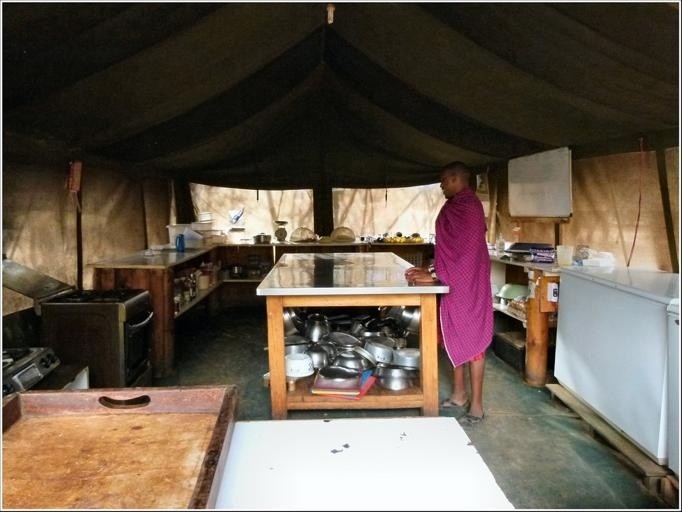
<box><xmin>508</xmin><ymin>146</ymin><xmax>573</xmax><ymax>218</ymax></box>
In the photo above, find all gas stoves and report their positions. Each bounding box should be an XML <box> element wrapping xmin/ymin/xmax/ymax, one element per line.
<box><xmin>1</xmin><ymin>346</ymin><xmax>61</xmax><ymax>397</ymax></box>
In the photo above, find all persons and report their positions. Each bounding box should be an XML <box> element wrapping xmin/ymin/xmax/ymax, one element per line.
<box><xmin>405</xmin><ymin>161</ymin><xmax>494</xmax><ymax>424</ymax></box>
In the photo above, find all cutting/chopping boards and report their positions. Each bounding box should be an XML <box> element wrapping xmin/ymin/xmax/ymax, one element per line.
<box><xmin>2</xmin><ymin>383</ymin><xmax>241</xmax><ymax>510</ymax></box>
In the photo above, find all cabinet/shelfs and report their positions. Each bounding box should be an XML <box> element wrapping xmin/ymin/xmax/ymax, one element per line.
<box><xmin>489</xmin><ymin>256</ymin><xmax>561</xmax><ymax>388</ymax></box>
<box><xmin>85</xmin><ymin>249</ymin><xmax>220</xmax><ymax>378</ymax></box>
<box><xmin>217</xmin><ymin>246</ymin><xmax>428</xmax><ymax>317</ymax></box>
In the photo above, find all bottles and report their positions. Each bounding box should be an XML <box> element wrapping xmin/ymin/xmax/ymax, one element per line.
<box><xmin>174</xmin><ymin>272</ymin><xmax>197</xmax><ymax>316</ymax></box>
<box><xmin>175</xmin><ymin>233</ymin><xmax>184</xmax><ymax>252</ymax></box>
<box><xmin>275</xmin><ymin>223</ymin><xmax>287</xmax><ymax>241</ymax></box>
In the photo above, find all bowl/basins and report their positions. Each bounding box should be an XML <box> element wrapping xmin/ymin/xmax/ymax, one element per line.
<box><xmin>496</xmin><ymin>284</ymin><xmax>530</xmax><ymax>300</ymax></box>
<box><xmin>283</xmin><ymin>306</ymin><xmax>422</xmax><ymax>392</ymax></box>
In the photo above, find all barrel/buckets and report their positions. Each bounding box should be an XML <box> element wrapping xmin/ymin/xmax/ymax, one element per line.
<box><xmin>166</xmin><ymin>224</ymin><xmax>192</xmax><ymax>243</ymax></box>
<box><xmin>191</xmin><ymin>222</ymin><xmax>212</xmax><ymax>230</ymax></box>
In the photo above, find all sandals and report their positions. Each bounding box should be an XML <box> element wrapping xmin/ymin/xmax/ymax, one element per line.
<box><xmin>458</xmin><ymin>413</ymin><xmax>485</xmax><ymax>431</ymax></box>
<box><xmin>439</xmin><ymin>398</ymin><xmax>471</xmax><ymax>412</ymax></box>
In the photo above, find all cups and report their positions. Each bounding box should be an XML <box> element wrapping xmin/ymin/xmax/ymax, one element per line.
<box><xmin>556</xmin><ymin>245</ymin><xmax>572</xmax><ymax>268</ymax></box>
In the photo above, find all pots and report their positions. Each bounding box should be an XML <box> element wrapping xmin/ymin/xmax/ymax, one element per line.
<box><xmin>253</xmin><ymin>233</ymin><xmax>271</xmax><ymax>245</ymax></box>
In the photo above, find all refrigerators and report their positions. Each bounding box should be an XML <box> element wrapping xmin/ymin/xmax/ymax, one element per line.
<box><xmin>553</xmin><ymin>266</ymin><xmax>679</xmax><ymax>479</ymax></box>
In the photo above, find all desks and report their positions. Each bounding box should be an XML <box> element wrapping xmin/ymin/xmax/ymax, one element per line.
<box><xmin>255</xmin><ymin>253</ymin><xmax>451</xmax><ymax>417</ymax></box>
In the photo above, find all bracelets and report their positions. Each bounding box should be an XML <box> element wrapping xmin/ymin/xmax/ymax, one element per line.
<box><xmin>431</xmin><ymin>272</ymin><xmax>437</xmax><ymax>280</ymax></box>
<box><xmin>429</xmin><ymin>264</ymin><xmax>434</xmax><ymax>272</ymax></box>
